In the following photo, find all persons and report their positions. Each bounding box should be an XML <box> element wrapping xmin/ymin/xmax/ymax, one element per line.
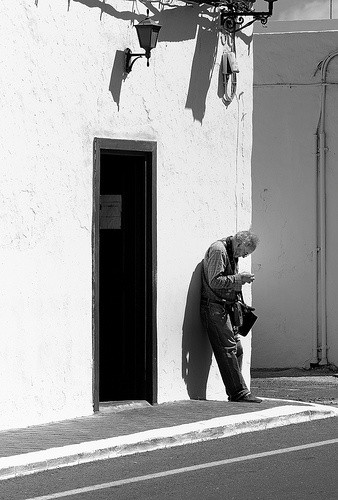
<box><xmin>201</xmin><ymin>231</ymin><xmax>263</xmax><ymax>403</ymax></box>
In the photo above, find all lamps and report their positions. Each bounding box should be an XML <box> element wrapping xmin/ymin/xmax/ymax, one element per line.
<box><xmin>123</xmin><ymin>9</ymin><xmax>162</xmax><ymax>73</ymax></box>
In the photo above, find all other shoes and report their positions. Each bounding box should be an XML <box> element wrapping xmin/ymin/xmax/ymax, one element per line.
<box><xmin>238</xmin><ymin>392</ymin><xmax>263</xmax><ymax>403</ymax></box>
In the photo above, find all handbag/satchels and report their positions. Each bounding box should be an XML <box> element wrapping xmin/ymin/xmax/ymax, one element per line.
<box><xmin>236</xmin><ymin>306</ymin><xmax>259</xmax><ymax>337</ymax></box>
